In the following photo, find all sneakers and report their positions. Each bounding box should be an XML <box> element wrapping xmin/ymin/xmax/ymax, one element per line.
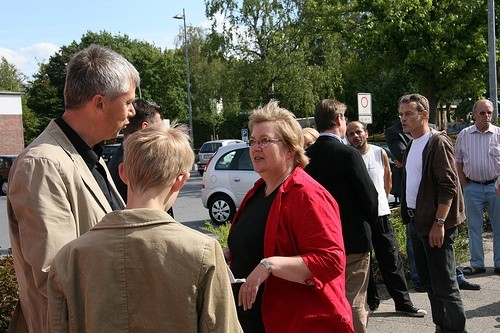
<box><xmin>396</xmin><ymin>305</ymin><xmax>428</xmax><ymax>317</ymax></box>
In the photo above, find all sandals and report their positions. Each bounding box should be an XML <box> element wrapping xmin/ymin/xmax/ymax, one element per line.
<box><xmin>462</xmin><ymin>266</ymin><xmax>486</xmax><ymax>275</ymax></box>
<box><xmin>493</xmin><ymin>267</ymin><xmax>500</xmax><ymax>274</ymax></box>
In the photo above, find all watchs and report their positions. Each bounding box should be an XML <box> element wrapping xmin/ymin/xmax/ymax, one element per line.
<box><xmin>434</xmin><ymin>217</ymin><xmax>445</xmax><ymax>225</ymax></box>
<box><xmin>260</xmin><ymin>258</ymin><xmax>271</xmax><ymax>272</ymax></box>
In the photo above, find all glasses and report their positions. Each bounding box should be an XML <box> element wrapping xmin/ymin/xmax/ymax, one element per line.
<box><xmin>249</xmin><ymin>136</ymin><xmax>286</xmax><ymax>146</ymax></box>
<box><xmin>398</xmin><ymin>111</ymin><xmax>414</xmax><ymax>118</ymax></box>
<box><xmin>337</xmin><ymin>112</ymin><xmax>348</xmax><ymax>122</ymax></box>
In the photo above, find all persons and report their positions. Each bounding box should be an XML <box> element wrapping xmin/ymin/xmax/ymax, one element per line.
<box><xmin>302</xmin><ymin>125</ymin><xmax>319</xmax><ymax>149</ymax></box>
<box><xmin>455</xmin><ymin>99</ymin><xmax>500</xmax><ymax>276</ymax></box>
<box><xmin>346</xmin><ymin>120</ymin><xmax>427</xmax><ymax>317</ymax></box>
<box><xmin>303</xmin><ymin>100</ymin><xmax>379</xmax><ymax>333</ymax></box>
<box><xmin>48</xmin><ymin>121</ymin><xmax>245</xmax><ymax>333</ymax></box>
<box><xmin>388</xmin><ymin>115</ymin><xmax>482</xmax><ymax>292</ymax></box>
<box><xmin>398</xmin><ymin>94</ymin><xmax>467</xmax><ymax>333</ymax></box>
<box><xmin>6</xmin><ymin>45</ymin><xmax>142</xmax><ymax>333</ymax></box>
<box><xmin>108</xmin><ymin>99</ymin><xmax>175</xmax><ymax>219</ymax></box>
<box><xmin>227</xmin><ymin>100</ymin><xmax>354</xmax><ymax>333</ymax></box>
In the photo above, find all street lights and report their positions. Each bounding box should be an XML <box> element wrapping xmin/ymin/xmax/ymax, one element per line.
<box><xmin>172</xmin><ymin>7</ymin><xmax>193</xmax><ymax>145</ymax></box>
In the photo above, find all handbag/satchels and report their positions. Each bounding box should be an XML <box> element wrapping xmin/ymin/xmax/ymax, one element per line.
<box><xmin>231</xmin><ymin>280</ymin><xmax>267</xmax><ymax>333</ymax></box>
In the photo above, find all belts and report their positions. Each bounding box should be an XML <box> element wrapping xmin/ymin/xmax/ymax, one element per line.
<box><xmin>407</xmin><ymin>208</ymin><xmax>416</xmax><ymax>219</ymax></box>
<box><xmin>466</xmin><ymin>177</ymin><xmax>496</xmax><ymax>185</ymax></box>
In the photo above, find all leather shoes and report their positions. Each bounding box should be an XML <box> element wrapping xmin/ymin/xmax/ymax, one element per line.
<box><xmin>458</xmin><ymin>281</ymin><xmax>480</xmax><ymax>290</ymax></box>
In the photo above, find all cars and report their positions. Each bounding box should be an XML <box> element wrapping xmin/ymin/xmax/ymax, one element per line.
<box><xmin>197</xmin><ymin>139</ymin><xmax>247</xmax><ymax>176</ymax></box>
<box><xmin>0</xmin><ymin>155</ymin><xmax>19</xmax><ymax>196</ymax></box>
<box><xmin>201</xmin><ymin>142</ymin><xmax>262</xmax><ymax>225</ymax></box>
